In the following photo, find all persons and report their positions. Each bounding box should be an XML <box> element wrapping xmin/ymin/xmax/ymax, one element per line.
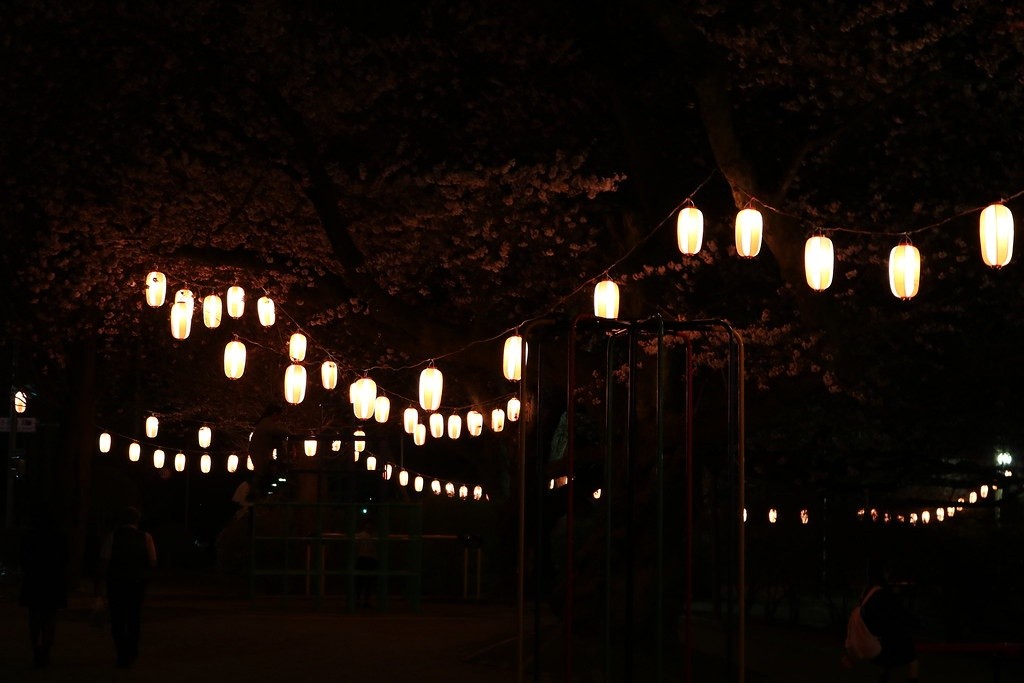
<box><xmin>99</xmin><ymin>507</ymin><xmax>157</xmax><ymax>669</ymax></box>
<box><xmin>858</xmin><ymin>559</ymin><xmax>930</xmax><ymax>683</ymax></box>
<box><xmin>241</xmin><ymin>403</ymin><xmax>291</xmax><ymax>503</ymax></box>
<box><xmin>19</xmin><ymin>505</ymin><xmax>70</xmax><ymax>671</ymax></box>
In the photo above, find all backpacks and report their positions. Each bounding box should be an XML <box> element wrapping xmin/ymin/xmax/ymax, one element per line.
<box><xmin>843</xmin><ymin>585</ymin><xmax>882</xmax><ymax>662</ymax></box>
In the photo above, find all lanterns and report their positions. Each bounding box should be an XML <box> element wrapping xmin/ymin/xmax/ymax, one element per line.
<box><xmin>15</xmin><ymin>203</ymin><xmax>1014</xmax><ymax>528</ymax></box>
